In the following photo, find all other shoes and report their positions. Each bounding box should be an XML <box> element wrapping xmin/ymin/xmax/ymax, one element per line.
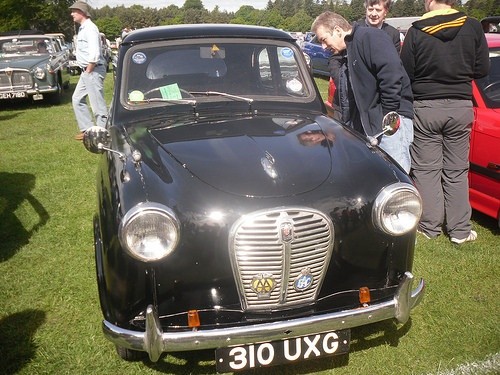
<box><xmin>76</xmin><ymin>133</ymin><xmax>84</xmax><ymax>140</ymax></box>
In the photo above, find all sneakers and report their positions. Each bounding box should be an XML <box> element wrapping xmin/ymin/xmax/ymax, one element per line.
<box><xmin>450</xmin><ymin>230</ymin><xmax>478</xmax><ymax>244</ymax></box>
<box><xmin>417</xmin><ymin>227</ymin><xmax>437</xmax><ymax>240</ymax></box>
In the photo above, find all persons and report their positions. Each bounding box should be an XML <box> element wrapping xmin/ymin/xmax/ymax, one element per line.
<box><xmin>400</xmin><ymin>0</ymin><xmax>490</xmax><ymax>244</ymax></box>
<box><xmin>309</xmin><ymin>0</ymin><xmax>413</xmax><ymax>176</ymax></box>
<box><xmin>37</xmin><ymin>42</ymin><xmax>49</xmax><ymax>53</ymax></box>
<box><xmin>115</xmin><ymin>24</ymin><xmax>135</xmax><ymax>49</ymax></box>
<box><xmin>62</xmin><ymin>1</ymin><xmax>108</xmax><ymax>141</ymax></box>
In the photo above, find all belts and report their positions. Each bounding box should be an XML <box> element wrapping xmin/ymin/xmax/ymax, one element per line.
<box><xmin>82</xmin><ymin>64</ymin><xmax>101</xmax><ymax>72</ymax></box>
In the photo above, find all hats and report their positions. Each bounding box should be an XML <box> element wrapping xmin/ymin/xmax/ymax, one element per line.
<box><xmin>68</xmin><ymin>1</ymin><xmax>92</xmax><ymax>18</ymax></box>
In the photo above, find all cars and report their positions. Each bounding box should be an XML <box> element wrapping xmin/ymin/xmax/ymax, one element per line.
<box><xmin>326</xmin><ymin>32</ymin><xmax>499</xmax><ymax>228</ymax></box>
<box><xmin>83</xmin><ymin>17</ymin><xmax>426</xmax><ymax>375</ymax></box>
<box><xmin>45</xmin><ymin>31</ymin><xmax>112</xmax><ymax>75</ymax></box>
<box><xmin>302</xmin><ymin>31</ymin><xmax>333</xmax><ymax>77</ymax></box>
<box><xmin>0</xmin><ymin>35</ymin><xmax>71</xmax><ymax>102</ymax></box>
